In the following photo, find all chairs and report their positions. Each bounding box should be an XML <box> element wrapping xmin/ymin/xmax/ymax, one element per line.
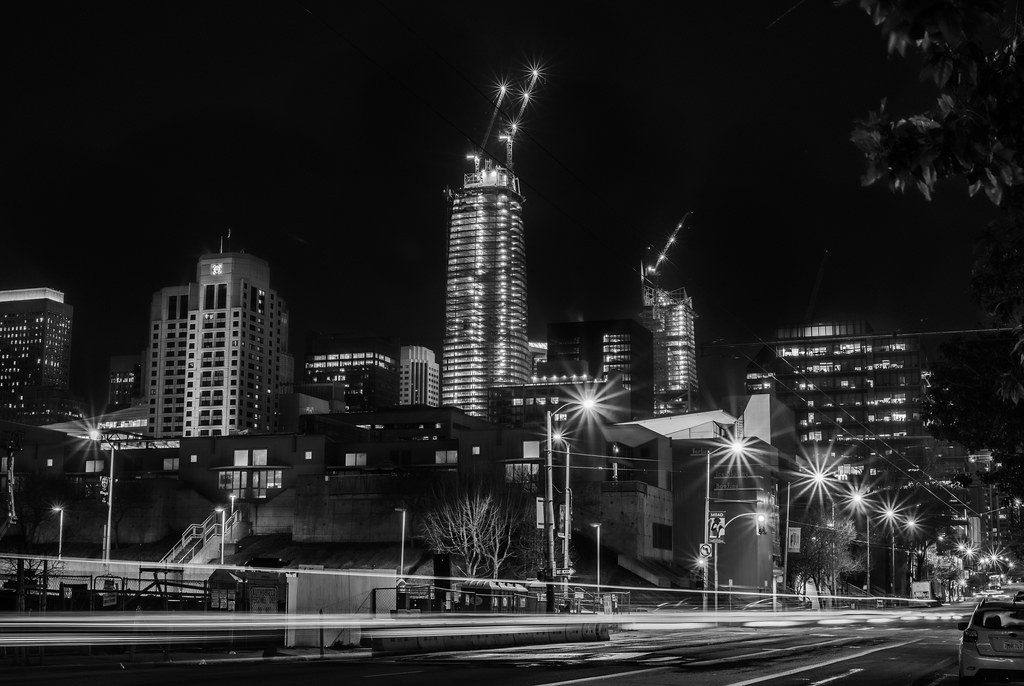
<box><xmin>984</xmin><ymin>614</ymin><xmax>1003</xmax><ymax>630</ymax></box>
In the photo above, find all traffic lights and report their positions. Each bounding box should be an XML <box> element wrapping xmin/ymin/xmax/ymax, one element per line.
<box><xmin>757</xmin><ymin>513</ymin><xmax>767</xmax><ymax>536</ymax></box>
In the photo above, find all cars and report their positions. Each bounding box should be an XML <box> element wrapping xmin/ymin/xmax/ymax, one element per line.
<box><xmin>972</xmin><ymin>586</ymin><xmax>1009</xmax><ymax>601</ymax></box>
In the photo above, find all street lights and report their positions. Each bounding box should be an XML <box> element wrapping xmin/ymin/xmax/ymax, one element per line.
<box><xmin>867</xmin><ymin>508</ymin><xmax>894</xmax><ymax>604</ymax></box>
<box><xmin>833</xmin><ymin>494</ymin><xmax>863</xmax><ymax>607</ymax></box>
<box><xmin>53</xmin><ymin>507</ymin><xmax>64</xmax><ymax>563</ymax></box>
<box><xmin>590</xmin><ymin>523</ymin><xmax>600</xmax><ymax>591</ymax></box>
<box><xmin>230</xmin><ymin>494</ymin><xmax>236</xmax><ymax>515</ymax></box>
<box><xmin>214</xmin><ymin>508</ymin><xmax>225</xmax><ymax>564</ymax></box>
<box><xmin>782</xmin><ymin>472</ymin><xmax>823</xmax><ymax>608</ymax></box>
<box><xmin>90</xmin><ymin>430</ymin><xmax>115</xmax><ymax>560</ymax></box>
<box><xmin>395</xmin><ymin>508</ymin><xmax>406</xmax><ymax>575</ymax></box>
<box><xmin>702</xmin><ymin>441</ymin><xmax>744</xmax><ymax>612</ymax></box>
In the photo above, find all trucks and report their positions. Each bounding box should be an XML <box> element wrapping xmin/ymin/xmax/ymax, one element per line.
<box><xmin>911</xmin><ymin>580</ymin><xmax>946</xmax><ymax>605</ymax></box>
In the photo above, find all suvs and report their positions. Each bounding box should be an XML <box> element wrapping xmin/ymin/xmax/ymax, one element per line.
<box><xmin>958</xmin><ymin>597</ymin><xmax>1024</xmax><ymax>681</ymax></box>
